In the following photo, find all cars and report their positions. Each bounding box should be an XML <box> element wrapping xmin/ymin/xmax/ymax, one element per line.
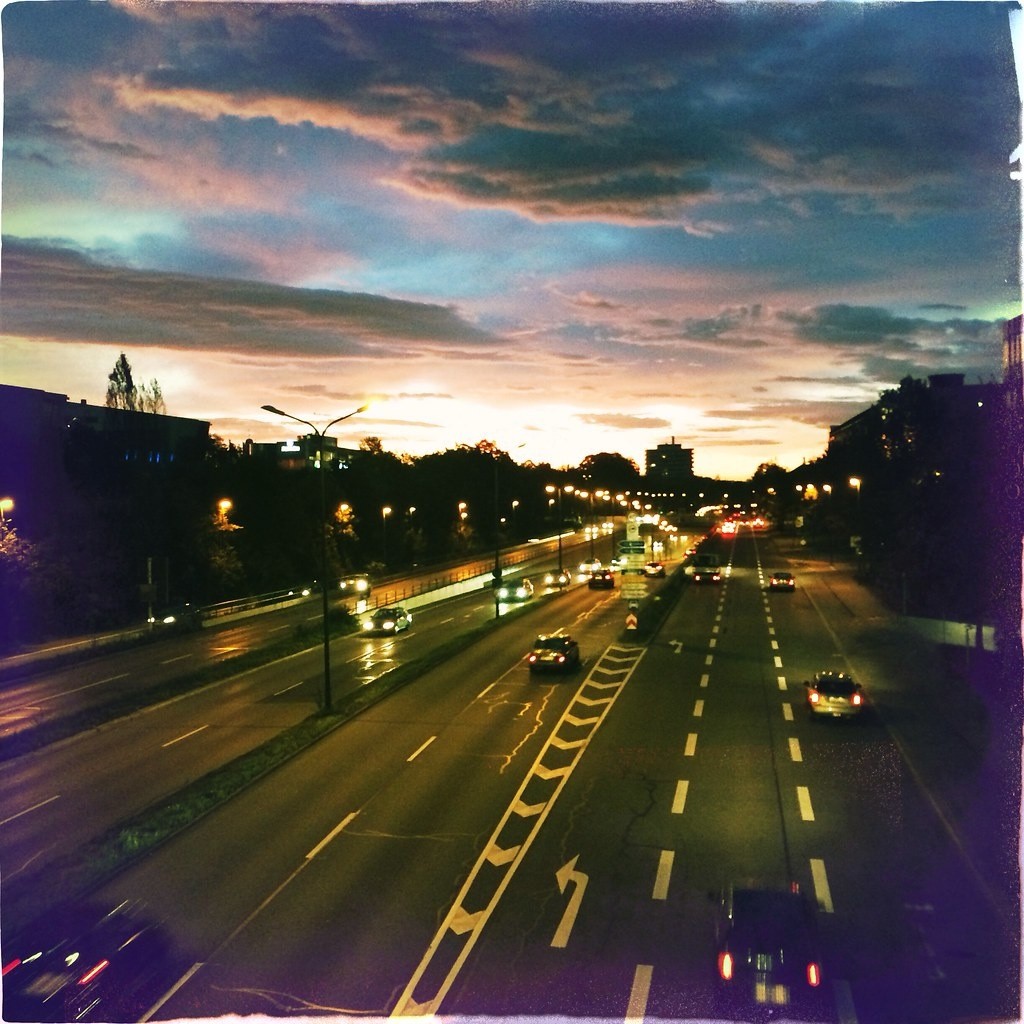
<box><xmin>587</xmin><ymin>568</ymin><xmax>615</xmax><ymax>590</ymax></box>
<box><xmin>528</xmin><ymin>632</ymin><xmax>580</xmax><ymax>672</ymax></box>
<box><xmin>704</xmin><ymin>882</ymin><xmax>826</xmax><ymax>1006</ymax></box>
<box><xmin>645</xmin><ymin>562</ymin><xmax>667</xmax><ymax>578</ymax></box>
<box><xmin>287</xmin><ymin>579</ymin><xmax>323</xmax><ymax>600</ymax></box>
<box><xmin>147</xmin><ymin>602</ymin><xmax>200</xmax><ymax>634</ymax></box>
<box><xmin>337</xmin><ymin>572</ymin><xmax>373</xmax><ymax>594</ymax></box>
<box><xmin>542</xmin><ymin>566</ymin><xmax>572</xmax><ymax>587</ymax></box>
<box><xmin>3</xmin><ymin>897</ymin><xmax>170</xmax><ymax>1023</ymax></box>
<box><xmin>802</xmin><ymin>670</ymin><xmax>863</xmax><ymax>722</ymax></box>
<box><xmin>767</xmin><ymin>571</ymin><xmax>796</xmax><ymax>592</ymax></box>
<box><xmin>363</xmin><ymin>605</ymin><xmax>414</xmax><ymax>635</ymax></box>
<box><xmin>579</xmin><ymin>557</ymin><xmax>602</xmax><ymax>575</ymax></box>
<box><xmin>498</xmin><ymin>577</ymin><xmax>535</xmax><ymax>603</ymax></box>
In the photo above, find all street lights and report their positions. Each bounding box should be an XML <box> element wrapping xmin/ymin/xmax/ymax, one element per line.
<box><xmin>0</xmin><ymin>496</ymin><xmax>16</xmax><ymax>547</ymax></box>
<box><xmin>602</xmin><ymin>492</ymin><xmax>626</xmax><ymax>559</ymax></box>
<box><xmin>216</xmin><ymin>497</ymin><xmax>234</xmax><ymax>552</ymax></box>
<box><xmin>579</xmin><ymin>488</ymin><xmax>605</xmax><ymax>563</ymax></box>
<box><xmin>382</xmin><ymin>505</ymin><xmax>393</xmax><ymax>573</ymax></box>
<box><xmin>336</xmin><ymin>501</ymin><xmax>352</xmax><ymax>565</ymax></box>
<box><xmin>847</xmin><ymin>477</ymin><xmax>863</xmax><ymax>528</ymax></box>
<box><xmin>259</xmin><ymin>397</ymin><xmax>389</xmax><ymax>710</ymax></box>
<box><xmin>459</xmin><ymin>439</ymin><xmax>529</xmax><ymax>620</ymax></box>
<box><xmin>543</xmin><ymin>482</ymin><xmax>574</xmax><ymax>573</ymax></box>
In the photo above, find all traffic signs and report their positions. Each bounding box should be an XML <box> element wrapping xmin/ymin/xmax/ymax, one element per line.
<box><xmin>618</xmin><ymin>547</ymin><xmax>645</xmax><ymax>555</ymax></box>
<box><xmin>615</xmin><ymin>540</ymin><xmax>645</xmax><ymax>548</ymax></box>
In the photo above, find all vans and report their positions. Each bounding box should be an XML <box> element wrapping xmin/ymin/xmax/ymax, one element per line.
<box><xmin>688</xmin><ymin>554</ymin><xmax>723</xmax><ymax>585</ymax></box>
